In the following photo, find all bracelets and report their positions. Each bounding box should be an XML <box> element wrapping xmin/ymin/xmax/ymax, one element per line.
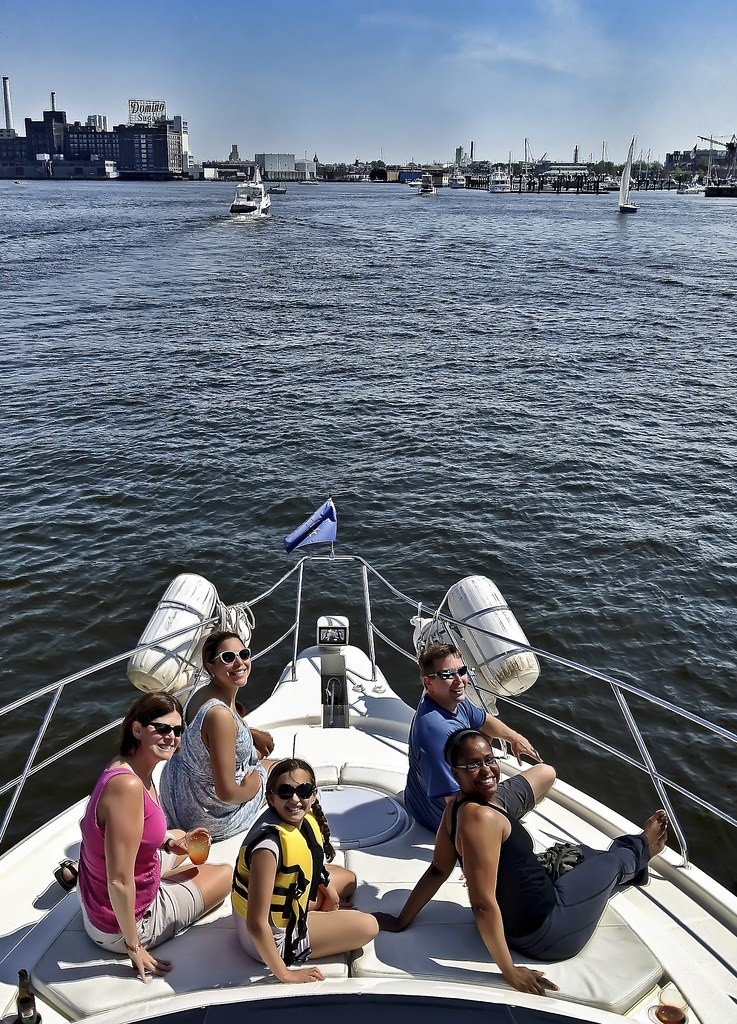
<box><xmin>124</xmin><ymin>938</ymin><xmax>141</xmax><ymax>954</ymax></box>
<box><xmin>164</xmin><ymin>838</ymin><xmax>173</xmax><ymax>854</ymax></box>
<box><xmin>54</xmin><ymin>692</ymin><xmax>233</xmax><ymax>984</ymax></box>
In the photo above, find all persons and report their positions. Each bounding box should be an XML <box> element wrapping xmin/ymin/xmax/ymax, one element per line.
<box><xmin>158</xmin><ymin>631</ymin><xmax>280</xmax><ymax>844</ymax></box>
<box><xmin>404</xmin><ymin>644</ymin><xmax>556</xmax><ymax>836</ymax></box>
<box><xmin>369</xmin><ymin>728</ymin><xmax>668</xmax><ymax>996</ymax></box>
<box><xmin>231</xmin><ymin>758</ymin><xmax>379</xmax><ymax>983</ymax></box>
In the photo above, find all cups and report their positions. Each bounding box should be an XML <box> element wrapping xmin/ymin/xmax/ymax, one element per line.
<box><xmin>185</xmin><ymin>826</ymin><xmax>210</xmax><ymax>865</ymax></box>
<box><xmin>657</xmin><ymin>989</ymin><xmax>689</xmax><ymax>1024</ymax></box>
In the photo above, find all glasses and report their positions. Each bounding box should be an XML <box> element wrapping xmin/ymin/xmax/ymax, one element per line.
<box><xmin>211</xmin><ymin>648</ymin><xmax>251</xmax><ymax>665</ymax></box>
<box><xmin>426</xmin><ymin>665</ymin><xmax>468</xmax><ymax>681</ymax></box>
<box><xmin>143</xmin><ymin>722</ymin><xmax>184</xmax><ymax>737</ymax></box>
<box><xmin>265</xmin><ymin>783</ymin><xmax>316</xmax><ymax>800</ymax></box>
<box><xmin>452</xmin><ymin>756</ymin><xmax>502</xmax><ymax>773</ymax></box>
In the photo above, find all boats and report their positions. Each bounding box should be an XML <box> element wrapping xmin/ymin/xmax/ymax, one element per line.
<box><xmin>267</xmin><ymin>182</ymin><xmax>288</xmax><ymax>194</ymax></box>
<box><xmin>229</xmin><ymin>168</ymin><xmax>272</xmax><ymax>218</ymax></box>
<box><xmin>448</xmin><ymin>172</ymin><xmax>466</xmax><ymax>188</ymax></box>
<box><xmin>489</xmin><ymin>167</ymin><xmax>511</xmax><ymax>193</ymax></box>
<box><xmin>408</xmin><ymin>178</ymin><xmax>422</xmax><ymax>187</ymax></box>
<box><xmin>418</xmin><ymin>173</ymin><xmax>436</xmax><ymax>195</ymax></box>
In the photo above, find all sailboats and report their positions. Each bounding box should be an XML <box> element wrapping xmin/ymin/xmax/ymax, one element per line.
<box><xmin>511</xmin><ymin>137</ymin><xmax>705</xmax><ymax>194</ymax></box>
<box><xmin>619</xmin><ymin>134</ymin><xmax>638</xmax><ymax>214</ymax></box>
<box><xmin>298</xmin><ymin>151</ymin><xmax>320</xmax><ymax>185</ymax></box>
<box><xmin>243</xmin><ymin>166</ymin><xmax>263</xmax><ymax>184</ymax></box>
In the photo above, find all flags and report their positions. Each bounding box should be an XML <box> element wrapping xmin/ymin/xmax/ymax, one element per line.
<box><xmin>283</xmin><ymin>498</ymin><xmax>337</xmax><ymax>555</ymax></box>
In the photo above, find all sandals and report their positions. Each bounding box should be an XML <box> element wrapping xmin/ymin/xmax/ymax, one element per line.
<box><xmin>55</xmin><ymin>860</ymin><xmax>79</xmax><ymax>892</ymax></box>
